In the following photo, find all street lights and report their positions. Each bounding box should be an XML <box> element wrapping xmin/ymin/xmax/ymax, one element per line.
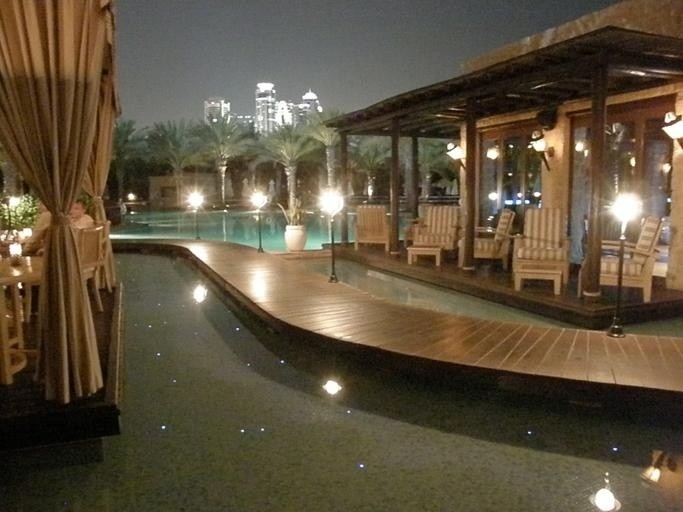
<box><xmin>606</xmin><ymin>194</ymin><xmax>642</xmax><ymax>337</ymax></box>
<box><xmin>318</xmin><ymin>189</ymin><xmax>345</xmax><ymax>283</ymax></box>
<box><xmin>188</xmin><ymin>192</ymin><xmax>202</xmax><ymax>239</ymax></box>
<box><xmin>250</xmin><ymin>191</ymin><xmax>268</xmax><ymax>253</ymax></box>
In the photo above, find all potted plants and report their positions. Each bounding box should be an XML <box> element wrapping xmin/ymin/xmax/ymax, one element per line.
<box><xmin>277</xmin><ymin>198</ymin><xmax>308</xmax><ymax>251</ymax></box>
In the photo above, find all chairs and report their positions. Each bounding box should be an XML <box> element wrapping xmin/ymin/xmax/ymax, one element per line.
<box><xmin>458</xmin><ymin>207</ymin><xmax>515</xmax><ymax>270</ymax></box>
<box><xmin>79</xmin><ymin>220</ymin><xmax>113</xmax><ymax>312</ymax></box>
<box><xmin>578</xmin><ymin>216</ymin><xmax>664</xmax><ymax>303</ymax></box>
<box><xmin>354</xmin><ymin>205</ymin><xmax>390</xmax><ymax>251</ymax></box>
<box><xmin>513</xmin><ymin>208</ymin><xmax>571</xmax><ymax>295</ymax></box>
<box><xmin>403</xmin><ymin>203</ymin><xmax>459</xmax><ymax>266</ymax></box>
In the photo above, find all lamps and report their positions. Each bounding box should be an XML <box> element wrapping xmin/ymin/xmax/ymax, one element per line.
<box><xmin>530</xmin><ymin>130</ymin><xmax>555</xmax><ymax>172</ymax></box>
<box><xmin>447</xmin><ymin>143</ymin><xmax>467</xmax><ymax>168</ymax></box>
<box><xmin>661</xmin><ymin>110</ymin><xmax>683</xmax><ymax>150</ymax></box>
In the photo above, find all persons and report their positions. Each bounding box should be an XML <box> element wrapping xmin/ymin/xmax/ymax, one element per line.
<box><xmin>70</xmin><ymin>199</ymin><xmax>94</xmax><ymax>228</ymax></box>
<box><xmin>32</xmin><ymin>202</ymin><xmax>51</xmax><ymax>229</ymax></box>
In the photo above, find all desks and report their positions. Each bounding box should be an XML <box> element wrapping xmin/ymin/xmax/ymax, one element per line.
<box><xmin>0</xmin><ymin>229</ymin><xmax>44</xmax><ymax>385</ymax></box>
